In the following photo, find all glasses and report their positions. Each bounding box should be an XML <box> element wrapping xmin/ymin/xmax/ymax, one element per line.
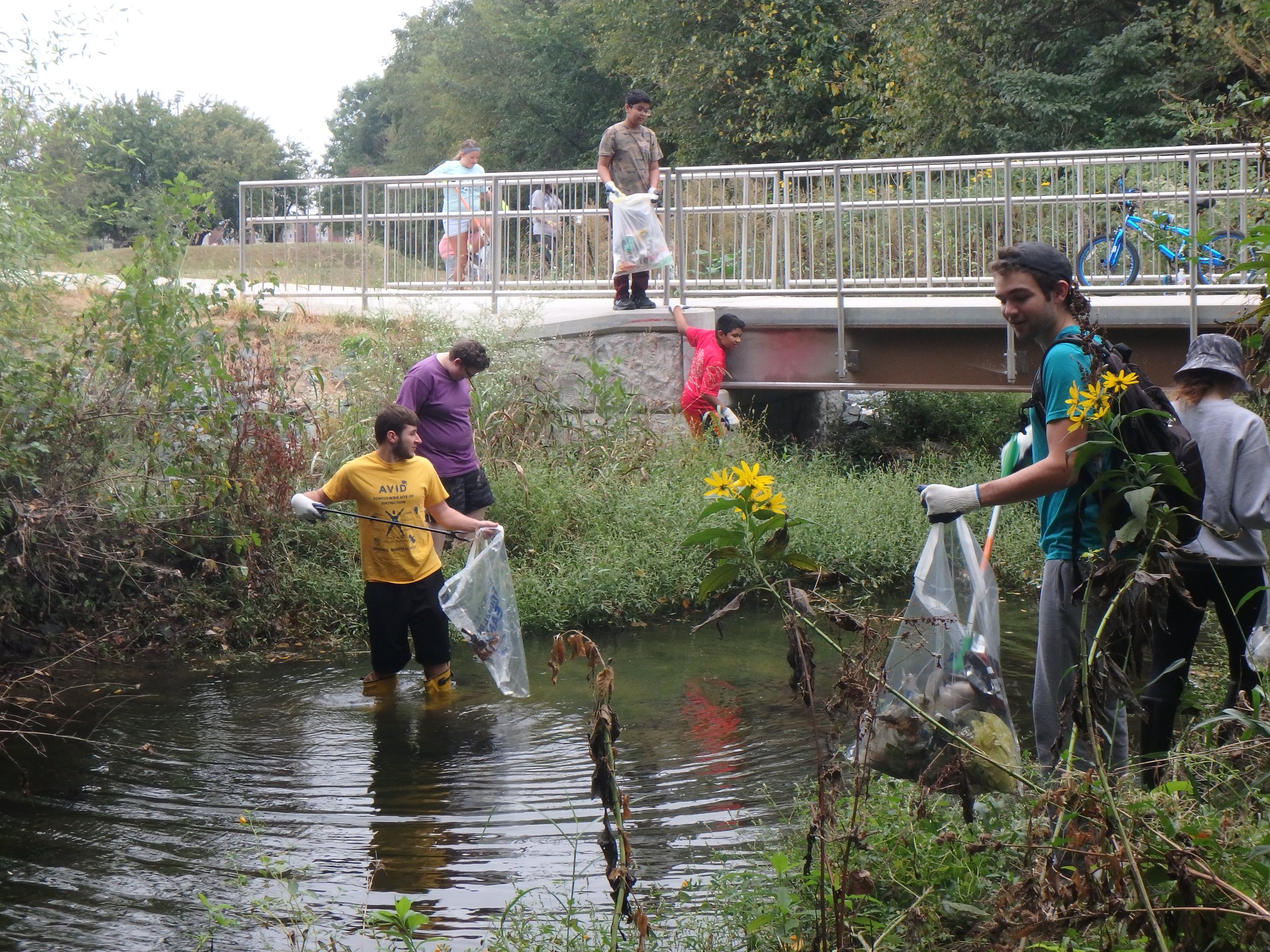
<box><xmin>628</xmin><ymin>104</ymin><xmax>651</xmax><ymax>116</ymax></box>
<box><xmin>461</xmin><ymin>361</ymin><xmax>480</xmax><ymax>377</ymax></box>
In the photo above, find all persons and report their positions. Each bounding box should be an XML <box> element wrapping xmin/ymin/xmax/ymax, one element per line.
<box><xmin>914</xmin><ymin>238</ymin><xmax>1148</xmax><ymax>798</ymax></box>
<box><xmin>1137</xmin><ymin>331</ymin><xmax>1270</xmax><ymax>791</ymax></box>
<box><xmin>396</xmin><ymin>339</ymin><xmax>491</xmax><ymax>559</ymax></box>
<box><xmin>528</xmin><ymin>182</ymin><xmax>565</xmax><ymax>279</ymax></box>
<box><xmin>439</xmin><ymin>230</ymin><xmax>492</xmax><ymax>284</ymax></box>
<box><xmin>288</xmin><ymin>404</ymin><xmax>499</xmax><ymax>695</ymax></box>
<box><xmin>666</xmin><ymin>303</ymin><xmax>746</xmax><ymax>459</ymax></box>
<box><xmin>597</xmin><ymin>89</ymin><xmax>665</xmax><ymax>311</ymax></box>
<box><xmin>423</xmin><ymin>139</ymin><xmax>512</xmax><ymax>290</ymax></box>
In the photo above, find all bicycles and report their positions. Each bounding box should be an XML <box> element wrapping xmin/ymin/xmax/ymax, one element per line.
<box><xmin>1075</xmin><ymin>166</ymin><xmax>1257</xmax><ymax>297</ymax></box>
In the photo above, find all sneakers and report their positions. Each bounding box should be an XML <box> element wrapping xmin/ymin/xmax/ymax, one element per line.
<box><xmin>630</xmin><ymin>293</ymin><xmax>656</xmax><ymax>309</ymax></box>
<box><xmin>613</xmin><ymin>297</ymin><xmax>637</xmax><ymax>310</ymax></box>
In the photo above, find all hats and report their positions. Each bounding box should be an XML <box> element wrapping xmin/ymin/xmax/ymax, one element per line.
<box><xmin>1006</xmin><ymin>241</ymin><xmax>1091</xmax><ymax>316</ymax></box>
<box><xmin>1173</xmin><ymin>333</ymin><xmax>1253</xmax><ymax>393</ymax></box>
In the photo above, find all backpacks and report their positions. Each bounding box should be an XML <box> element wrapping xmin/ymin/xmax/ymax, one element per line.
<box><xmin>1014</xmin><ymin>334</ymin><xmax>1210</xmax><ymax>598</ymax></box>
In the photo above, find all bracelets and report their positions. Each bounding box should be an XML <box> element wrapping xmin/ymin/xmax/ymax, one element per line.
<box><xmin>442</xmin><ymin>180</ymin><xmax>449</xmax><ymax>182</ymax></box>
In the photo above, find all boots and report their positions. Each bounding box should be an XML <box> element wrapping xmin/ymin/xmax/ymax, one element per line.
<box><xmin>423</xmin><ymin>668</ymin><xmax>454</xmax><ymax>692</ymax></box>
<box><xmin>360</xmin><ymin>672</ymin><xmax>398</xmax><ymax>695</ymax></box>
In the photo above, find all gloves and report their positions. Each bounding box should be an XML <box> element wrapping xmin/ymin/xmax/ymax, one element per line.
<box><xmin>290</xmin><ymin>493</ymin><xmax>327</xmax><ymax>525</ymax></box>
<box><xmin>668</xmin><ymin>303</ymin><xmax>689</xmax><ymax>315</ymax></box>
<box><xmin>604</xmin><ymin>181</ymin><xmax>660</xmax><ymax>204</ymax></box>
<box><xmin>721</xmin><ymin>407</ymin><xmax>740</xmax><ymax>432</ymax></box>
<box><xmin>1000</xmin><ymin>424</ymin><xmax>1032</xmax><ymax>473</ymax></box>
<box><xmin>917</xmin><ymin>483</ymin><xmax>982</xmax><ymax>524</ymax></box>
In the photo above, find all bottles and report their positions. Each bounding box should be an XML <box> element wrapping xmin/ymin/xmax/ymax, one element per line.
<box><xmin>1177</xmin><ymin>268</ymin><xmax>1186</xmax><ymax>296</ymax></box>
